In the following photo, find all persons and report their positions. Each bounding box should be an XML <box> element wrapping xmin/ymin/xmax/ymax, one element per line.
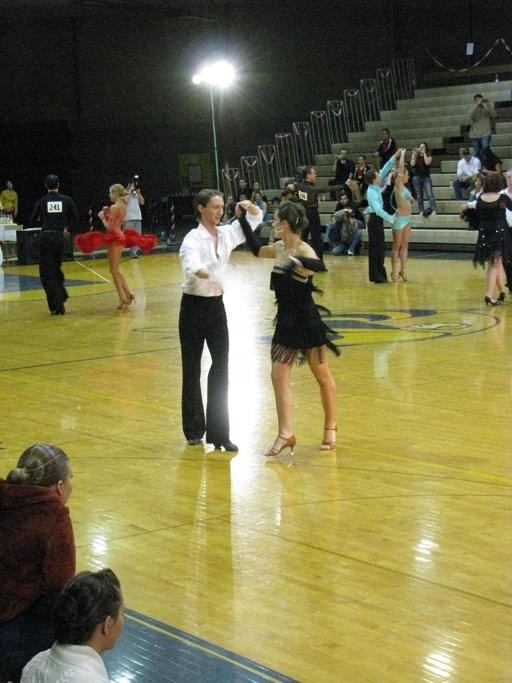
<box><xmin>323</xmin><ymin>147</ymin><xmax>373</xmax><ymax>256</ymax></box>
<box><xmin>0</xmin><ymin>442</ymin><xmax>76</xmax><ymax>625</ymax></box>
<box><xmin>364</xmin><ymin>148</ymin><xmax>403</xmax><ymax>284</ymax></box>
<box><xmin>235</xmin><ymin>178</ymin><xmax>269</xmax><ymax>248</ymax></box>
<box><xmin>31</xmin><ymin>175</ymin><xmax>80</xmax><ymax>315</ymax></box>
<box><xmin>453</xmin><ymin>149</ymin><xmax>483</xmax><ymax>201</ymax></box>
<box><xmin>467</xmin><ymin>147</ymin><xmax>503</xmax><ymax>201</ymax></box>
<box><xmin>460</xmin><ymin>169</ymin><xmax>512</xmax><ymax>304</ymax></box>
<box><xmin>265</xmin><ymin>167</ymin><xmax>354</xmax><ymax>272</ymax></box>
<box><xmin>237</xmin><ymin>199</ymin><xmax>344</xmax><ymax>457</ymax></box>
<box><xmin>1</xmin><ymin>181</ymin><xmax>18</xmax><ymax>219</ymax></box>
<box><xmin>76</xmin><ymin>183</ymin><xmax>157</xmax><ymax>309</ymax></box>
<box><xmin>375</xmin><ymin>142</ymin><xmax>438</xmax><ymax>217</ymax></box>
<box><xmin>468</xmin><ymin>94</ymin><xmax>498</xmax><ymax>166</ymax></box>
<box><xmin>373</xmin><ymin>128</ymin><xmax>397</xmax><ymax>172</ymax></box>
<box><xmin>390</xmin><ymin>148</ymin><xmax>415</xmax><ymax>281</ymax></box>
<box><xmin>122</xmin><ymin>183</ymin><xmax>145</xmax><ymax>257</ymax></box>
<box><xmin>178</xmin><ymin>189</ymin><xmax>264</xmax><ymax>451</ymax></box>
<box><xmin>19</xmin><ymin>568</ymin><xmax>123</xmax><ymax>682</ymax></box>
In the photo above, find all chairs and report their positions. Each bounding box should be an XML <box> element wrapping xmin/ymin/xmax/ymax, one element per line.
<box><xmin>1</xmin><ymin>223</ymin><xmax>24</xmax><ymax>264</ymax></box>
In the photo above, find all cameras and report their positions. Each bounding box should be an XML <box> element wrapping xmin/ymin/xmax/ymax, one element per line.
<box><xmin>131</xmin><ymin>175</ymin><xmax>140</xmax><ymax>191</ymax></box>
<box><xmin>416</xmin><ymin>148</ymin><xmax>422</xmax><ymax>152</ymax></box>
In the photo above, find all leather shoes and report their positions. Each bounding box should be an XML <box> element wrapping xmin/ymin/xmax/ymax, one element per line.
<box><xmin>214</xmin><ymin>440</ymin><xmax>238</xmax><ymax>450</ymax></box>
<box><xmin>188</xmin><ymin>437</ymin><xmax>200</xmax><ymax>443</ymax></box>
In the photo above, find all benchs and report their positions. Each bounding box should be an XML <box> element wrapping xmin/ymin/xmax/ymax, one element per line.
<box><xmin>248</xmin><ymin>73</ymin><xmax>512</xmax><ymax>243</ymax></box>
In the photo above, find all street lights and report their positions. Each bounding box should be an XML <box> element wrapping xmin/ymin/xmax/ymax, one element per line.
<box><xmin>187</xmin><ymin>55</ymin><xmax>238</xmax><ymax>193</ymax></box>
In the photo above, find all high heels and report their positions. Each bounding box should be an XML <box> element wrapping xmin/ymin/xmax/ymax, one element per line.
<box><xmin>116</xmin><ymin>298</ymin><xmax>129</xmax><ymax>309</ymax></box>
<box><xmin>498</xmin><ymin>292</ymin><xmax>505</xmax><ymax>300</ymax></box>
<box><xmin>391</xmin><ymin>272</ymin><xmax>399</xmax><ymax>282</ymax></box>
<box><xmin>399</xmin><ymin>271</ymin><xmax>409</xmax><ymax>280</ymax></box>
<box><xmin>264</xmin><ymin>433</ymin><xmax>296</xmax><ymax>455</ymax></box>
<box><xmin>486</xmin><ymin>295</ymin><xmax>501</xmax><ymax>305</ymax></box>
<box><xmin>320</xmin><ymin>425</ymin><xmax>337</xmax><ymax>450</ymax></box>
<box><xmin>127</xmin><ymin>292</ymin><xmax>136</xmax><ymax>305</ymax></box>
